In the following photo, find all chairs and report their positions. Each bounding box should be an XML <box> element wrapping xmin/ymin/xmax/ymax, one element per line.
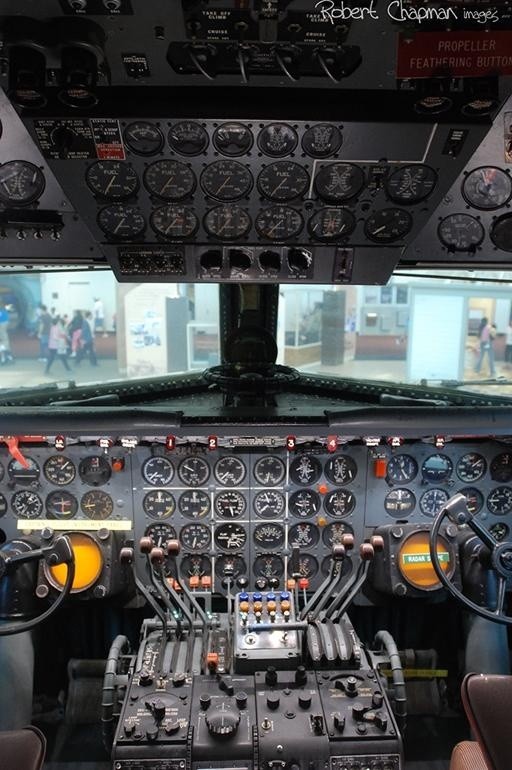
<box><xmin>0</xmin><ymin>725</ymin><xmax>47</xmax><ymax>769</ymax></box>
<box><xmin>452</xmin><ymin>672</ymin><xmax>511</xmax><ymax>770</ymax></box>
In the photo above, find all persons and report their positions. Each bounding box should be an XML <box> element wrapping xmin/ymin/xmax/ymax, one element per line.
<box><xmin>0</xmin><ymin>306</ymin><xmax>15</xmax><ymax>367</ymax></box>
<box><xmin>483</xmin><ymin>323</ymin><xmax>496</xmax><ymax>349</ymax></box>
<box><xmin>502</xmin><ymin>321</ymin><xmax>511</xmax><ymax>364</ymax></box>
<box><xmin>477</xmin><ymin>317</ymin><xmax>496</xmax><ymax>377</ymax></box>
<box><xmin>34</xmin><ymin>293</ymin><xmax>106</xmax><ymax>375</ymax></box>
